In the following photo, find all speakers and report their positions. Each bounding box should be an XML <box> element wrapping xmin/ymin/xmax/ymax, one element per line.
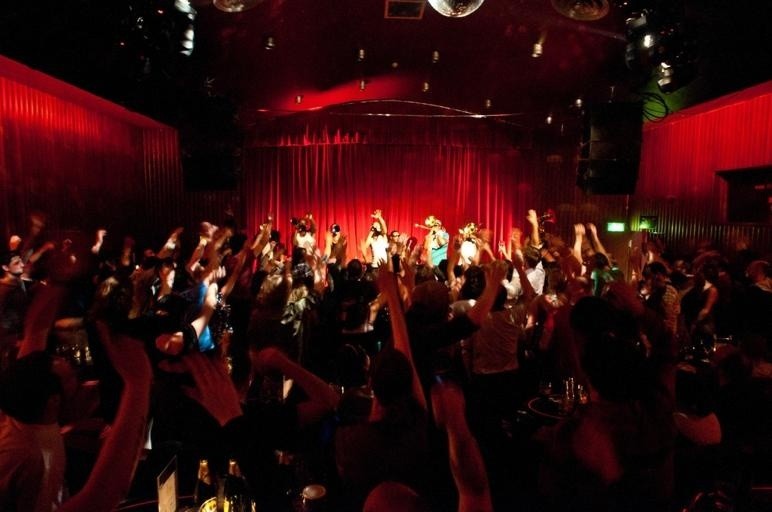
<box><xmin>179</xmin><ymin>97</ymin><xmax>238</xmax><ymax>192</ymax></box>
<box><xmin>575</xmin><ymin>100</ymin><xmax>643</xmax><ymax>196</ymax></box>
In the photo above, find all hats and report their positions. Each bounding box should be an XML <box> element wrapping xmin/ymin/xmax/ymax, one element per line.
<box><xmin>155</xmin><ymin>211</ymin><xmax>668</xmax><ymax>320</ymax></box>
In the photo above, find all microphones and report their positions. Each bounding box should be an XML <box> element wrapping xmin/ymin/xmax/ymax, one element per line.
<box><xmin>371</xmin><ymin>226</ymin><xmax>377</xmax><ymax>231</ymax></box>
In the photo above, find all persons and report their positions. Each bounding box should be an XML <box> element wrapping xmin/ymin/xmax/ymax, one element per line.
<box><xmin>0</xmin><ymin>209</ymin><xmax>772</xmax><ymax>512</ymax></box>
